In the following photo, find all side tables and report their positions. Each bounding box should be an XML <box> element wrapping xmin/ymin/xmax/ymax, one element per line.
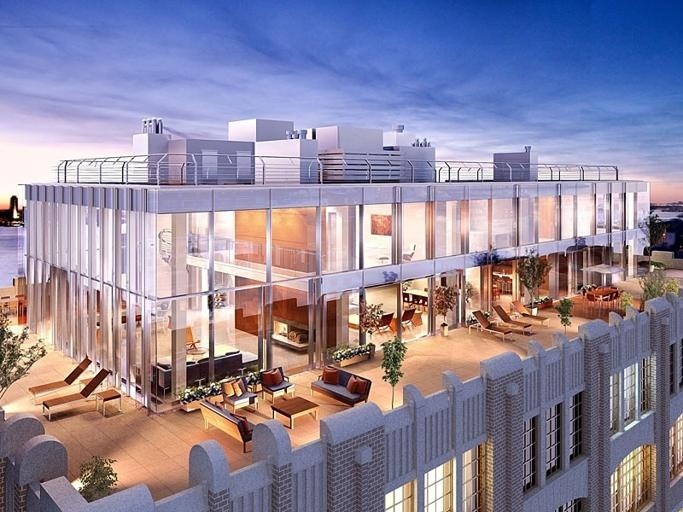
<box><xmin>95</xmin><ymin>389</ymin><xmax>121</xmax><ymax>416</ymax></box>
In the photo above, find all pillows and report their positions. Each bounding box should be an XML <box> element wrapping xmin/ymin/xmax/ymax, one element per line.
<box><xmin>261</xmin><ymin>368</ymin><xmax>282</xmax><ymax>388</ymax></box>
<box><xmin>370</xmin><ymin>307</ymin><xmax>416</xmax><ymax>337</ymax></box>
<box><xmin>321</xmin><ymin>366</ymin><xmax>338</xmax><ymax>385</ymax></box>
<box><xmin>345</xmin><ymin>375</ymin><xmax>367</xmax><ymax>395</ymax></box>
<box><xmin>214</xmin><ymin>401</ymin><xmax>248</xmax><ymax>434</ymax></box>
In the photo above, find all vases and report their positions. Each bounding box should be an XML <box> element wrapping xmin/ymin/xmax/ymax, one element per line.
<box><xmin>331</xmin><ymin>352</ymin><xmax>370</xmax><ymax>368</ymax></box>
<box><xmin>179</xmin><ymin>397</ymin><xmax>209</xmax><ymax>413</ymax></box>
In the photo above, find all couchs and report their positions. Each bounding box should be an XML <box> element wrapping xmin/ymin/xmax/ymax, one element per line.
<box><xmin>310</xmin><ymin>365</ymin><xmax>371</xmax><ymax>407</ymax></box>
<box><xmin>199</xmin><ymin>399</ymin><xmax>256</xmax><ymax>453</ymax></box>
<box><xmin>151</xmin><ymin>349</ymin><xmax>242</xmax><ymax>396</ymax></box>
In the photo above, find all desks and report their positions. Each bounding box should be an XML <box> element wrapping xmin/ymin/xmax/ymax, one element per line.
<box><xmin>241</xmin><ymin>351</ymin><xmax>258</xmax><ymax>376</ymax></box>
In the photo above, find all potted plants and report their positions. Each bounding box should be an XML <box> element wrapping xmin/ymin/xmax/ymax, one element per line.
<box><xmin>637</xmin><ymin>214</ymin><xmax>667</xmax><ymax>273</ymax></box>
<box><xmin>431</xmin><ymin>286</ymin><xmax>460</xmax><ymax>337</ymax></box>
<box><xmin>514</xmin><ymin>254</ymin><xmax>552</xmax><ymax>317</ymax></box>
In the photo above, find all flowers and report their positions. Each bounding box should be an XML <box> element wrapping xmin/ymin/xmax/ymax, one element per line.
<box><xmin>175</xmin><ymin>368</ymin><xmax>266</xmax><ymax>405</ymax></box>
<box><xmin>331</xmin><ymin>343</ymin><xmax>371</xmax><ymax>363</ymax></box>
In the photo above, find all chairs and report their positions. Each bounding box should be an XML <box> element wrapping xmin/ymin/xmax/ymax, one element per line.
<box><xmin>220</xmin><ymin>376</ymin><xmax>258</xmax><ymax>414</ymax></box>
<box><xmin>582</xmin><ymin>286</ymin><xmax>624</xmax><ymax>312</ymax></box>
<box><xmin>468</xmin><ymin>300</ymin><xmax>550</xmax><ymax>343</ymax></box>
<box><xmin>402</xmin><ymin>292</ymin><xmax>428</xmax><ymax>312</ymax></box>
<box><xmin>259</xmin><ymin>366</ymin><xmax>295</xmax><ymax>404</ymax></box>
<box><xmin>185</xmin><ymin>327</ymin><xmax>200</xmax><ymax>349</ymax></box>
<box><xmin>27</xmin><ymin>357</ymin><xmax>110</xmax><ymax>421</ymax></box>
